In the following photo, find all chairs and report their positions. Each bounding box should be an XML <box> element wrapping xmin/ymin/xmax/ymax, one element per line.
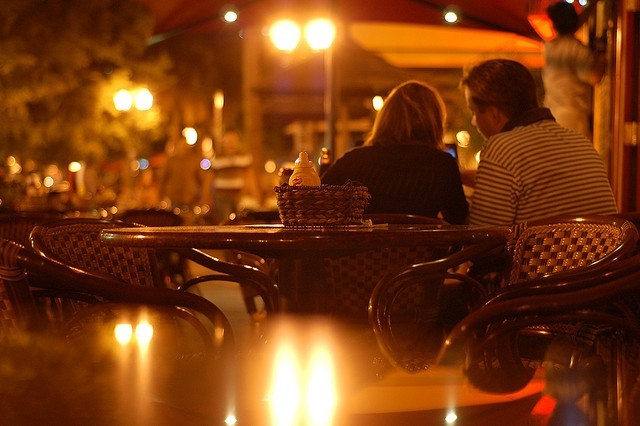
<box><xmin>0</xmin><ymin>240</ymin><xmax>236</xmax><ymax>372</ymax></box>
<box><xmin>28</xmin><ymin>217</ymin><xmax>281</xmax><ymax>356</ymax></box>
<box><xmin>368</xmin><ymin>213</ymin><xmax>637</xmax><ymax>375</ymax></box>
<box><xmin>292</xmin><ymin>213</ymin><xmax>464</xmax><ymax>322</ymax></box>
<box><xmin>439</xmin><ymin>254</ymin><xmax>640</xmax><ymax>426</ymax></box>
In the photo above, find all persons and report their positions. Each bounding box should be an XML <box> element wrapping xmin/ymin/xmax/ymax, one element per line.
<box><xmin>320</xmin><ymin>80</ymin><xmax>470</xmax><ymax>225</ymax></box>
<box><xmin>541</xmin><ymin>0</ymin><xmax>608</xmax><ymax>144</ymax></box>
<box><xmin>445</xmin><ymin>58</ymin><xmax>617</xmax><ymax>284</ymax></box>
